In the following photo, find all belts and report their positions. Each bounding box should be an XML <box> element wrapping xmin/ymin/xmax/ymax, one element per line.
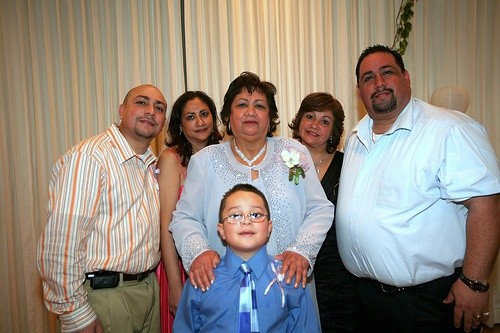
<box><xmin>86</xmin><ymin>268</ymin><xmax>156</xmax><ymax>282</ymax></box>
<box><xmin>378</xmin><ymin>275</ymin><xmax>456</xmax><ymax>293</ymax></box>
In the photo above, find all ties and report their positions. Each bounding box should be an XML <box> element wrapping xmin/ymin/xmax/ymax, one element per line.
<box><xmin>239</xmin><ymin>262</ymin><xmax>259</xmax><ymax>333</ymax></box>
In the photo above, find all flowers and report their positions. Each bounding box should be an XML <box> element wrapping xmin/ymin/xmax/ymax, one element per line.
<box><xmin>281</xmin><ymin>149</ymin><xmax>306</xmax><ymax>185</ymax></box>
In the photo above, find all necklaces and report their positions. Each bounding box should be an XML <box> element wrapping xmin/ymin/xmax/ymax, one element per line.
<box><xmin>191</xmin><ymin>142</ymin><xmax>202</xmax><ymax>151</ymax></box>
<box><xmin>233</xmin><ymin>137</ymin><xmax>266</xmax><ymax>166</ymax></box>
<box><xmin>372</xmin><ymin>130</ymin><xmax>376</xmax><ymax>144</ymax></box>
<box><xmin>314</xmin><ymin>160</ymin><xmax>322</xmax><ymax>173</ymax></box>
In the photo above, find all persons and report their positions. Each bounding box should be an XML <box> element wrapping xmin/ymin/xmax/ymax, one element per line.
<box><xmin>168</xmin><ymin>71</ymin><xmax>335</xmax><ymax>323</ymax></box>
<box><xmin>152</xmin><ymin>90</ymin><xmax>224</xmax><ymax>332</ymax></box>
<box><xmin>36</xmin><ymin>84</ymin><xmax>167</xmax><ymax>333</ymax></box>
<box><xmin>172</xmin><ymin>183</ymin><xmax>321</xmax><ymax>333</ymax></box>
<box><xmin>289</xmin><ymin>91</ymin><xmax>356</xmax><ymax>333</ymax></box>
<box><xmin>334</xmin><ymin>44</ymin><xmax>500</xmax><ymax>333</ymax></box>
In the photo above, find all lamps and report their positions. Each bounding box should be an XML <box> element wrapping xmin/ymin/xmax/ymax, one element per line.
<box><xmin>431</xmin><ymin>86</ymin><xmax>470</xmax><ymax>114</ymax></box>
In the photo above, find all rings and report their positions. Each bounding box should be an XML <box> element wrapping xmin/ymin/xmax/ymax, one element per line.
<box><xmin>482</xmin><ymin>312</ymin><xmax>489</xmax><ymax>319</ymax></box>
<box><xmin>473</xmin><ymin>315</ymin><xmax>482</xmax><ymax>321</ymax></box>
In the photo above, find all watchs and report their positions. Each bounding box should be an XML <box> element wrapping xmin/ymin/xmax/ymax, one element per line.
<box><xmin>459</xmin><ymin>274</ymin><xmax>489</xmax><ymax>292</ymax></box>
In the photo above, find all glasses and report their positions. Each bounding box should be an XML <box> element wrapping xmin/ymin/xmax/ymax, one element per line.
<box><xmin>221</xmin><ymin>212</ymin><xmax>268</xmax><ymax>224</ymax></box>
